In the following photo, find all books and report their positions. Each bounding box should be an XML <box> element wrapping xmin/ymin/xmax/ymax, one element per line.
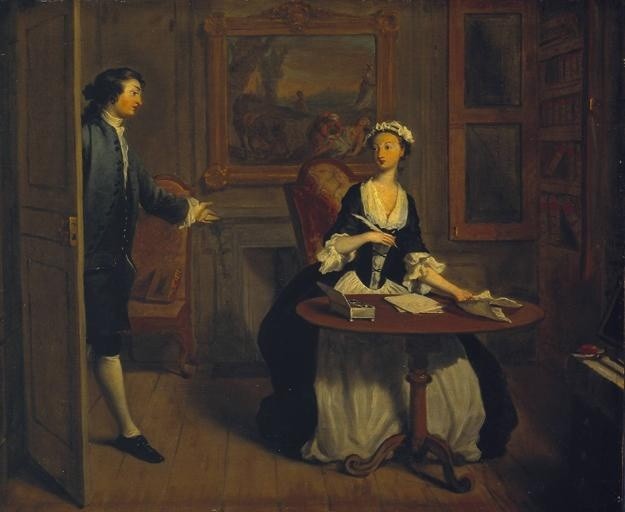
<box><xmin>383</xmin><ymin>293</ymin><xmax>445</xmax><ymax>314</ymax></box>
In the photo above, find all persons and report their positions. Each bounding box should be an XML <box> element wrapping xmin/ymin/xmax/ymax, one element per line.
<box><xmin>80</xmin><ymin>64</ymin><xmax>221</xmax><ymax>462</ymax></box>
<box><xmin>297</xmin><ymin>119</ymin><xmax>487</xmax><ymax>465</ymax></box>
